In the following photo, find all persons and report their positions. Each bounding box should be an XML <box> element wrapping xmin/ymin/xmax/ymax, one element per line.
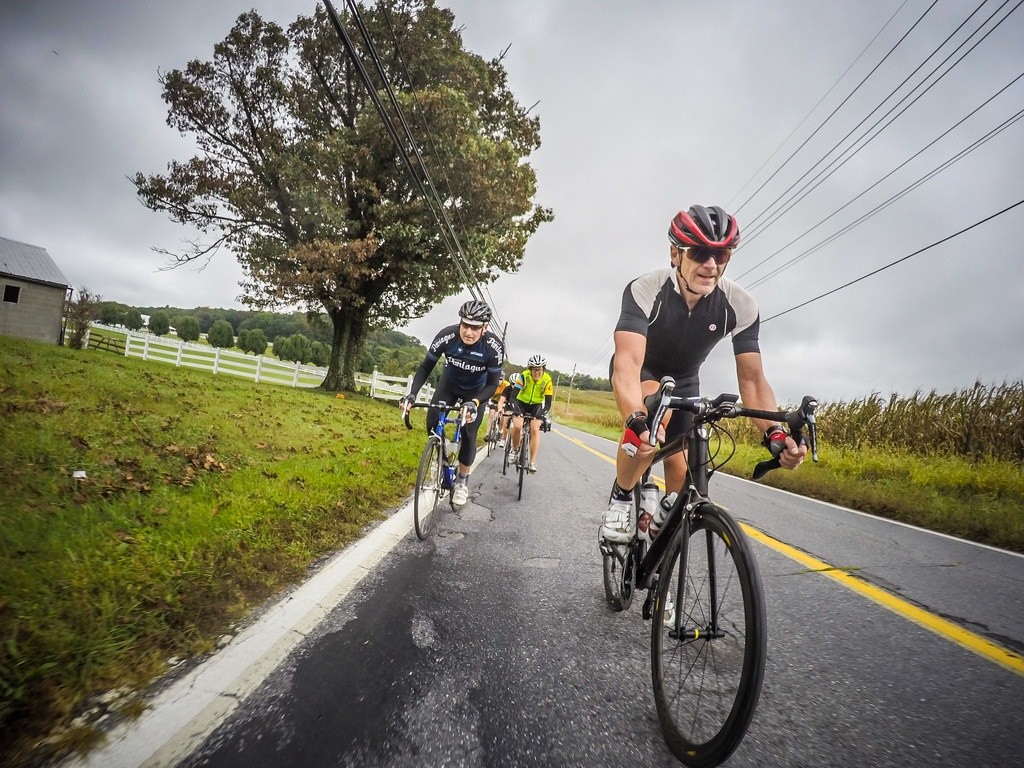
<box><xmin>484</xmin><ymin>371</ymin><xmax>510</xmax><ymax>447</ymax></box>
<box><xmin>601</xmin><ymin>206</ymin><xmax>809</xmax><ymax>628</ymax></box>
<box><xmin>507</xmin><ymin>354</ymin><xmax>553</xmax><ymax>474</ymax></box>
<box><xmin>498</xmin><ymin>373</ymin><xmax>520</xmax><ymax>448</ymax></box>
<box><xmin>399</xmin><ymin>301</ymin><xmax>505</xmax><ymax>506</ymax></box>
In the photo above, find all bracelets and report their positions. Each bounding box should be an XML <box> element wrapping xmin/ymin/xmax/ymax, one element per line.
<box><xmin>472</xmin><ymin>399</ymin><xmax>479</xmax><ymax>407</ymax></box>
<box><xmin>624</xmin><ymin>411</ymin><xmax>646</xmax><ymax>428</ymax></box>
<box><xmin>763</xmin><ymin>425</ymin><xmax>788</xmax><ymax>440</ymax></box>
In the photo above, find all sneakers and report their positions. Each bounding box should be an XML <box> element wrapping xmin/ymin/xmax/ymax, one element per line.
<box><xmin>498</xmin><ymin>439</ymin><xmax>505</xmax><ymax>447</ymax></box>
<box><xmin>647</xmin><ymin>585</ymin><xmax>677</xmax><ymax>628</ymax></box>
<box><xmin>507</xmin><ymin>448</ymin><xmax>519</xmax><ymax>464</ymax></box>
<box><xmin>430</xmin><ymin>455</ymin><xmax>438</xmax><ymax>481</ymax></box>
<box><xmin>529</xmin><ymin>463</ymin><xmax>536</xmax><ymax>475</ymax></box>
<box><xmin>451</xmin><ymin>484</ymin><xmax>468</xmax><ymax>506</ymax></box>
<box><xmin>601</xmin><ymin>491</ymin><xmax>634</xmax><ymax>543</ymax></box>
<box><xmin>483</xmin><ymin>434</ymin><xmax>489</xmax><ymax>442</ymax></box>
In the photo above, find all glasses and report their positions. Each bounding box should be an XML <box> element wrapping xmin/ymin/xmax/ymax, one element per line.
<box><xmin>512</xmin><ymin>383</ymin><xmax>515</xmax><ymax>385</ymax></box>
<box><xmin>676</xmin><ymin>246</ymin><xmax>732</xmax><ymax>265</ymax></box>
<box><xmin>499</xmin><ymin>378</ymin><xmax>503</xmax><ymax>381</ymax></box>
<box><xmin>461</xmin><ymin>321</ymin><xmax>483</xmax><ymax>331</ymax></box>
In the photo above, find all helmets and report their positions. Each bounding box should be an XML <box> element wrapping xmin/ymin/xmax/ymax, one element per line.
<box><xmin>500</xmin><ymin>371</ymin><xmax>505</xmax><ymax>377</ymax></box>
<box><xmin>527</xmin><ymin>354</ymin><xmax>547</xmax><ymax>369</ymax></box>
<box><xmin>509</xmin><ymin>372</ymin><xmax>520</xmax><ymax>383</ymax></box>
<box><xmin>458</xmin><ymin>300</ymin><xmax>492</xmax><ymax>326</ymax></box>
<box><xmin>668</xmin><ymin>203</ymin><xmax>741</xmax><ymax>250</ymax></box>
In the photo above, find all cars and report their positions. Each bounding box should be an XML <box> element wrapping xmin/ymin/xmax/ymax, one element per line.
<box><xmin>540</xmin><ymin>401</ymin><xmax>552</xmax><ymax>432</ymax></box>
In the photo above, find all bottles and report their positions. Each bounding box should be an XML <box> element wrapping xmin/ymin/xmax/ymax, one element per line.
<box><xmin>636</xmin><ymin>476</ymin><xmax>661</xmax><ymax>541</ymax></box>
<box><xmin>447</xmin><ymin>438</ymin><xmax>458</xmax><ymax>465</ymax></box>
<box><xmin>645</xmin><ymin>492</ymin><xmax>679</xmax><ymax>545</ymax></box>
<box><xmin>444</xmin><ymin>438</ymin><xmax>451</xmax><ymax>458</ymax></box>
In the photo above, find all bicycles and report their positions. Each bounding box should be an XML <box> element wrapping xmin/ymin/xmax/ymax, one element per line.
<box><xmin>487</xmin><ymin>400</ymin><xmax>547</xmax><ymax>501</ymax></box>
<box><xmin>401</xmin><ymin>398</ymin><xmax>477</xmax><ymax>542</ymax></box>
<box><xmin>597</xmin><ymin>376</ymin><xmax>818</xmax><ymax>768</ymax></box>
